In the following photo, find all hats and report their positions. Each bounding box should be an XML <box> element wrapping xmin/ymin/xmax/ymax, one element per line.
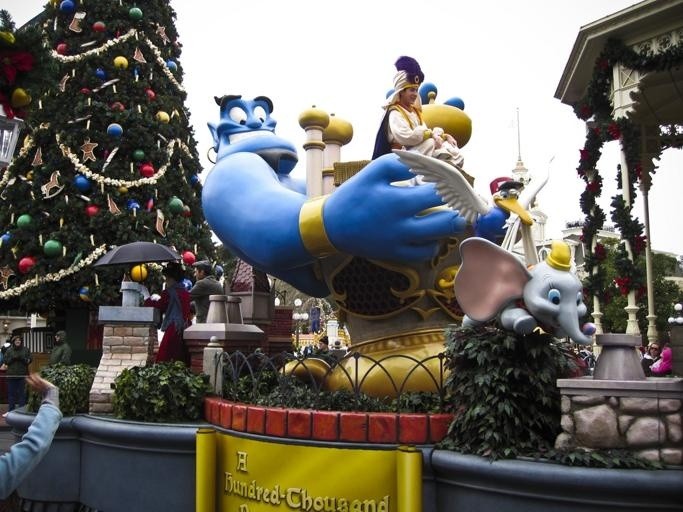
<box><xmin>380</xmin><ymin>68</ymin><xmax>425</xmax><ymax>91</ymax></box>
<box><xmin>192</xmin><ymin>259</ymin><xmax>212</xmax><ymax>274</ymax></box>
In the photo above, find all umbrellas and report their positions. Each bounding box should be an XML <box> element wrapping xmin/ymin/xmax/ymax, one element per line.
<box><xmin>92</xmin><ymin>241</ymin><xmax>183</xmax><ymax>268</ymax></box>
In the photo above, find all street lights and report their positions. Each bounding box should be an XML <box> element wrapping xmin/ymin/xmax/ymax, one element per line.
<box><xmin>267</xmin><ymin>277</ymin><xmax>280</xmax><ymax>306</ymax></box>
<box><xmin>291</xmin><ymin>298</ymin><xmax>308</xmax><ymax>353</ymax></box>
<box><xmin>667</xmin><ymin>303</ymin><xmax>683</xmax><ymax>326</ymax></box>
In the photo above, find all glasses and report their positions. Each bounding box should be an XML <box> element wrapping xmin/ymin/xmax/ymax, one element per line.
<box><xmin>649</xmin><ymin>347</ymin><xmax>660</xmax><ymax>352</ymax></box>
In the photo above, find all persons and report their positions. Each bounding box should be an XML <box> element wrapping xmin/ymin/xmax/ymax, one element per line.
<box><xmin>144</xmin><ymin>259</ymin><xmax>225</xmax><ymax>369</ymax></box>
<box><xmin>561</xmin><ymin>341</ymin><xmax>674</xmax><ymax>378</ymax></box>
<box><xmin>0</xmin><ymin>331</ymin><xmax>73</xmax><ymax>512</ymax></box>
<box><xmin>385</xmin><ymin>56</ymin><xmax>465</xmax><ymax>168</ymax></box>
<box><xmin>298</xmin><ymin>335</ymin><xmax>349</xmax><ymax>368</ymax></box>
<box><xmin>309</xmin><ymin>300</ymin><xmax>321</xmax><ymax>334</ymax></box>
